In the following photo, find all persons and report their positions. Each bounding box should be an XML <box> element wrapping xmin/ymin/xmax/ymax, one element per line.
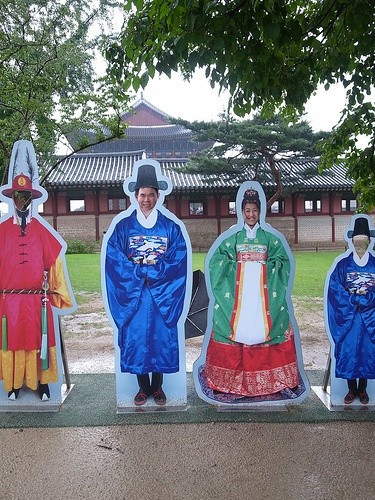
<box><xmin>105</xmin><ymin>164</ymin><xmax>187</xmax><ymax>405</ymax></box>
<box><xmin>327</xmin><ymin>217</ymin><xmax>375</xmax><ymax>405</ymax></box>
<box><xmin>0</xmin><ymin>172</ymin><xmax>74</xmax><ymax>401</ymax></box>
<box><xmin>198</xmin><ymin>187</ymin><xmax>306</xmax><ymax>404</ymax></box>
